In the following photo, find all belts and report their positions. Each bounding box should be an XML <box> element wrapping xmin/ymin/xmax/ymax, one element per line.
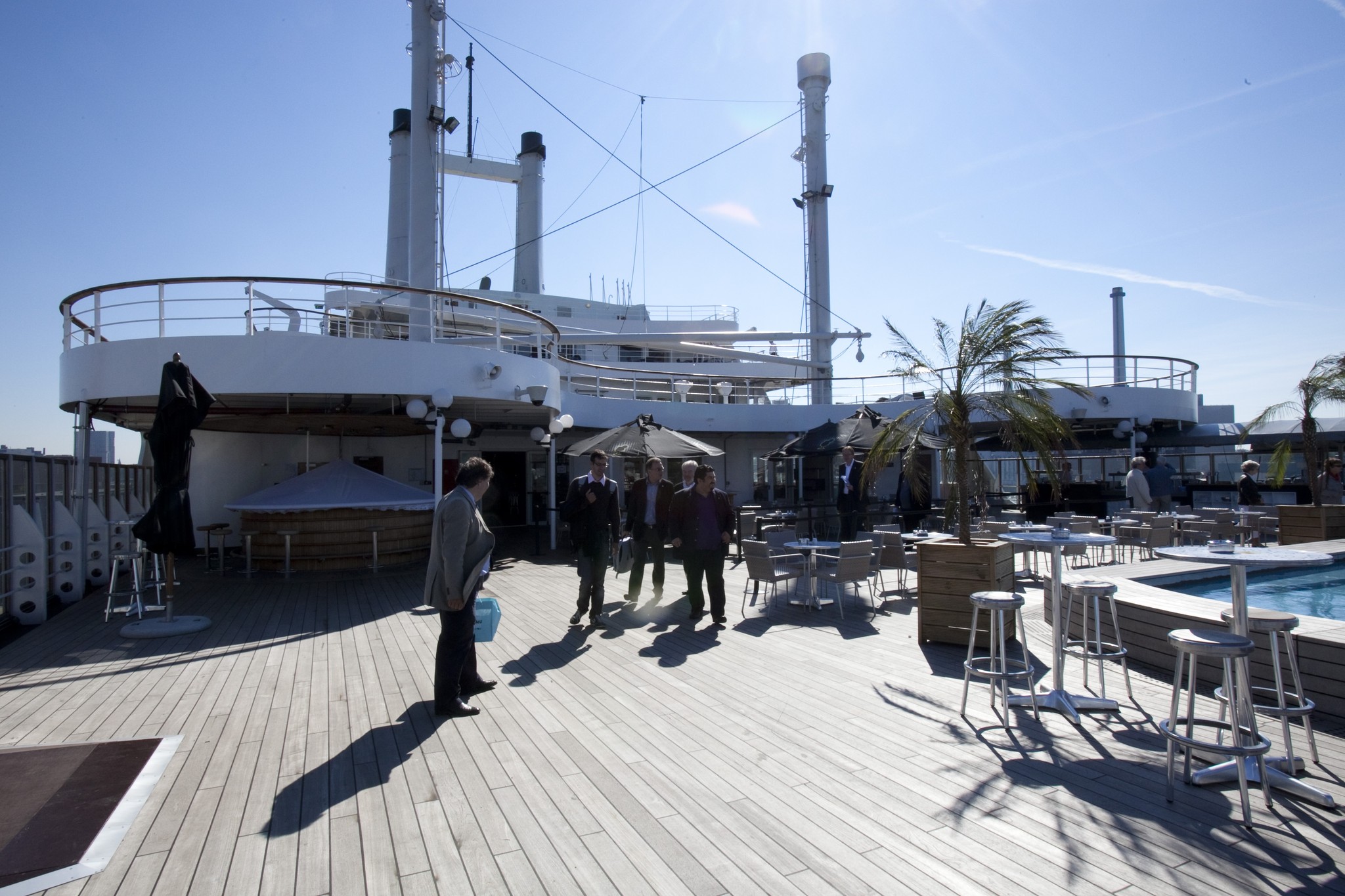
<box><xmin>643</xmin><ymin>523</ymin><xmax>657</xmax><ymax>529</ymax></box>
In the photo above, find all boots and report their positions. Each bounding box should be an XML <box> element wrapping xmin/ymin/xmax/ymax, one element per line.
<box><xmin>1251</xmin><ymin>538</ymin><xmax>1265</xmax><ymax>547</ymax></box>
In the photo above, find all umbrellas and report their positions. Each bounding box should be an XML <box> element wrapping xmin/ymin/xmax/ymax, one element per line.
<box><xmin>554</xmin><ymin>413</ymin><xmax>726</xmax><ymax>479</ymax></box>
<box><xmin>131</xmin><ymin>353</ymin><xmax>216</xmax><ymax>620</ymax></box>
<box><xmin>758</xmin><ymin>405</ymin><xmax>955</xmax><ymax>461</ymax></box>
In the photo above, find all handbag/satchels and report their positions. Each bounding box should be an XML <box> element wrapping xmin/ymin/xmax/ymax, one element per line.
<box><xmin>473</xmin><ymin>593</ymin><xmax>502</xmax><ymax>642</ymax></box>
<box><xmin>613</xmin><ymin>533</ymin><xmax>635</xmax><ymax>573</ymax></box>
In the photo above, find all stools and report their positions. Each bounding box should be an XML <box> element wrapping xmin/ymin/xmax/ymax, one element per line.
<box><xmin>961</xmin><ymin>591</ymin><xmax>1040</xmax><ymax>729</ymax></box>
<box><xmin>1063</xmin><ymin>581</ymin><xmax>1133</xmax><ymax>699</ymax></box>
<box><xmin>365</xmin><ymin>526</ymin><xmax>385</xmax><ymax>574</ymax></box>
<box><xmin>105</xmin><ymin>552</ymin><xmax>144</xmax><ymax>623</ymax></box>
<box><xmin>557</xmin><ymin>522</ymin><xmax>570</xmax><ymax>542</ymax></box>
<box><xmin>1167</xmin><ymin>610</ymin><xmax>1319</xmax><ymax>827</ymax></box>
<box><xmin>197</xmin><ymin>523</ymin><xmax>299</xmax><ymax>575</ymax></box>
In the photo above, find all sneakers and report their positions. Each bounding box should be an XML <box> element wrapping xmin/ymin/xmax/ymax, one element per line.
<box><xmin>590</xmin><ymin>615</ymin><xmax>606</xmax><ymax>628</ymax></box>
<box><xmin>570</xmin><ymin>610</ymin><xmax>587</xmax><ymax>625</ymax></box>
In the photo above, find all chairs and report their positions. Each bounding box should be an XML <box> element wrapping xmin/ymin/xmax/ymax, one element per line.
<box><xmin>733</xmin><ymin>506</ymin><xmax>1280</xmax><ymax>619</ymax></box>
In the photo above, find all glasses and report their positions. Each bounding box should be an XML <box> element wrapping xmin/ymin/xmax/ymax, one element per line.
<box><xmin>1334</xmin><ymin>465</ymin><xmax>1341</xmax><ymax>467</ymax></box>
<box><xmin>842</xmin><ymin>453</ymin><xmax>852</xmax><ymax>457</ymax></box>
<box><xmin>594</xmin><ymin>462</ymin><xmax>609</xmax><ymax>468</ymax></box>
<box><xmin>649</xmin><ymin>467</ymin><xmax>665</xmax><ymax>472</ymax></box>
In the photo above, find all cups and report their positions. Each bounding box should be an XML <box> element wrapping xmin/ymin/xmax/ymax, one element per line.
<box><xmin>1166</xmin><ymin>511</ymin><xmax>1177</xmax><ymax>516</ymax></box>
<box><xmin>787</xmin><ymin>510</ymin><xmax>789</xmax><ymax>513</ymax></box>
<box><xmin>790</xmin><ymin>510</ymin><xmax>793</xmax><ymax>514</ymax></box>
<box><xmin>1029</xmin><ymin>521</ymin><xmax>1033</xmax><ymax>527</ymax></box>
<box><xmin>1025</xmin><ymin>521</ymin><xmax>1029</xmax><ymax>527</ymax></box>
<box><xmin>812</xmin><ymin>538</ymin><xmax>818</xmax><ymax>544</ymax></box>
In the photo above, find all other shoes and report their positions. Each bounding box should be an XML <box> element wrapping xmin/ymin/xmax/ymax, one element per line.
<box><xmin>624</xmin><ymin>594</ymin><xmax>638</xmax><ymax>602</ymax></box>
<box><xmin>682</xmin><ymin>590</ymin><xmax>688</xmax><ymax>594</ymax></box>
<box><xmin>654</xmin><ymin>594</ymin><xmax>663</xmax><ymax>599</ymax></box>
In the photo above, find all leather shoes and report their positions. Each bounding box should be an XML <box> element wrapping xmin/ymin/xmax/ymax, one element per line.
<box><xmin>689</xmin><ymin>610</ymin><xmax>703</xmax><ymax>619</ymax></box>
<box><xmin>475</xmin><ymin>680</ymin><xmax>497</xmax><ymax>691</ymax></box>
<box><xmin>713</xmin><ymin>616</ymin><xmax>727</xmax><ymax>623</ymax></box>
<box><xmin>448</xmin><ymin>702</ymin><xmax>481</xmax><ymax>716</ymax></box>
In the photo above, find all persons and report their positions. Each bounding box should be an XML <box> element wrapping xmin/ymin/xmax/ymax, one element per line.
<box><xmin>896</xmin><ymin>471</ymin><xmax>932</xmax><ymax>552</ymax></box>
<box><xmin>1237</xmin><ymin>459</ymin><xmax>1268</xmax><ymax>548</ymax></box>
<box><xmin>836</xmin><ymin>445</ymin><xmax>869</xmax><ymax>543</ymax></box>
<box><xmin>1317</xmin><ymin>457</ymin><xmax>1343</xmax><ymax>505</ymax></box>
<box><xmin>1058</xmin><ymin>463</ymin><xmax>1072</xmax><ymax>481</ymax></box>
<box><xmin>423</xmin><ymin>457</ymin><xmax>496</xmax><ymax>716</ymax></box>
<box><xmin>1145</xmin><ymin>456</ymin><xmax>1177</xmax><ymax>514</ymax></box>
<box><xmin>624</xmin><ymin>458</ymin><xmax>735</xmax><ymax>623</ymax></box>
<box><xmin>562</xmin><ymin>449</ymin><xmax>620</xmax><ymax>628</ymax></box>
<box><xmin>1126</xmin><ymin>457</ymin><xmax>1153</xmax><ymax>512</ymax></box>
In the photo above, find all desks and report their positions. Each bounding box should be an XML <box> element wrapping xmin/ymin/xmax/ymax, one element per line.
<box><xmin>105</xmin><ymin>512</ymin><xmax>181</xmax><ymax>616</ymax></box>
<box><xmin>1153</xmin><ymin>548</ymin><xmax>1335</xmax><ymax>808</ymax></box>
<box><xmin>1159</xmin><ymin>515</ymin><xmax>1202</xmax><ymax>547</ymax></box>
<box><xmin>1098</xmin><ymin>519</ymin><xmax>1139</xmax><ymax>567</ymax></box>
<box><xmin>1234</xmin><ymin>511</ymin><xmax>1268</xmax><ymax>547</ymax></box>
<box><xmin>783</xmin><ymin>541</ymin><xmax>841</xmax><ymax>610</ymax></box>
<box><xmin>1009</xmin><ymin>525</ymin><xmax>1054</xmax><ymax>578</ymax></box>
<box><xmin>901</xmin><ymin>532</ymin><xmax>953</xmax><ymax>540</ymax></box>
<box><xmin>766</xmin><ymin>513</ymin><xmax>797</xmax><ymax>528</ymax></box>
<box><xmin>998</xmin><ymin>533</ymin><xmax>1119</xmax><ymax>724</ymax></box>
